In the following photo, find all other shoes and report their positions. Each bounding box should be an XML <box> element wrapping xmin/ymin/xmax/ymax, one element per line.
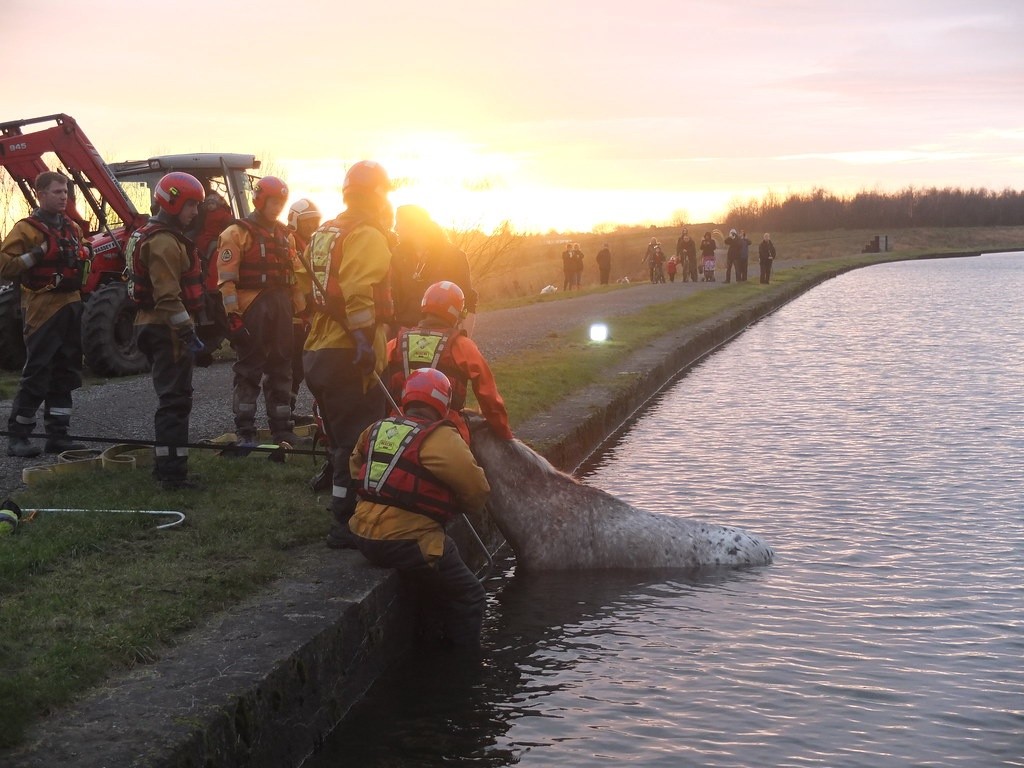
<box><xmin>162</xmin><ymin>472</ymin><xmax>200</xmax><ymax>489</ymax></box>
<box><xmin>309</xmin><ymin>459</ymin><xmax>332</xmax><ymax>489</ymax></box>
<box><xmin>232</xmin><ymin>423</ymin><xmax>258</xmax><ymax>444</ymax></box>
<box><xmin>44</xmin><ymin>437</ymin><xmax>87</xmax><ymax>453</ymax></box>
<box><xmin>721</xmin><ymin>280</ymin><xmax>730</xmax><ymax>284</ymax></box>
<box><xmin>328</xmin><ymin>522</ymin><xmax>361</xmax><ymax>548</ymax></box>
<box><xmin>271</xmin><ymin>428</ymin><xmax>312</xmax><ymax>446</ymax></box>
<box><xmin>6</xmin><ymin>435</ymin><xmax>41</xmax><ymax>457</ymax></box>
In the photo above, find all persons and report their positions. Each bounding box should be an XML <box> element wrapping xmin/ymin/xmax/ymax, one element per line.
<box><xmin>182</xmin><ymin>176</ymin><xmax>235</xmax><ymax>323</ymax></box>
<box><xmin>385</xmin><ymin>280</ymin><xmax>515</xmax><ymax>443</ymax></box>
<box><xmin>562</xmin><ymin>242</ymin><xmax>584</xmax><ymax>291</ymax></box>
<box><xmin>123</xmin><ymin>171</ymin><xmax>207</xmax><ymax>492</ymax></box>
<box><xmin>597</xmin><ymin>243</ymin><xmax>611</xmax><ymax>285</ymax></box>
<box><xmin>639</xmin><ymin>228</ymin><xmax>776</xmax><ymax>284</ymax></box>
<box><xmin>302</xmin><ymin>160</ymin><xmax>397</xmax><ymax>550</ymax></box>
<box><xmin>349</xmin><ymin>366</ymin><xmax>490</xmax><ymax>606</ymax></box>
<box><xmin>285</xmin><ymin>198</ymin><xmax>321</xmax><ymax>427</ymax></box>
<box><xmin>1</xmin><ymin>171</ymin><xmax>95</xmax><ymax>459</ymax></box>
<box><xmin>396</xmin><ymin>204</ymin><xmax>478</xmax><ymax>328</ymax></box>
<box><xmin>216</xmin><ymin>177</ymin><xmax>313</xmax><ymax>457</ymax></box>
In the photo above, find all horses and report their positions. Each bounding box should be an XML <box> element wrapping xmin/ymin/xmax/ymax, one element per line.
<box><xmin>458</xmin><ymin>408</ymin><xmax>775</xmax><ymax>572</ymax></box>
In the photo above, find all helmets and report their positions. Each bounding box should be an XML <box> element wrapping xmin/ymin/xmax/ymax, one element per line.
<box><xmin>421</xmin><ymin>280</ymin><xmax>465</xmax><ymax>327</ymax></box>
<box><xmin>401</xmin><ymin>367</ymin><xmax>453</xmax><ymax>419</ymax></box>
<box><xmin>341</xmin><ymin>160</ymin><xmax>396</xmax><ymax>203</ymax></box>
<box><xmin>253</xmin><ymin>177</ymin><xmax>288</xmax><ymax>209</ymax></box>
<box><xmin>153</xmin><ymin>172</ymin><xmax>205</xmax><ymax>215</ymax></box>
<box><xmin>287</xmin><ymin>198</ymin><xmax>321</xmax><ymax>231</ymax></box>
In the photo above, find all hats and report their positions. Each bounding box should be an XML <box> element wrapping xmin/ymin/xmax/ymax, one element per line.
<box><xmin>207</xmin><ymin>194</ymin><xmax>221</xmax><ymax>205</ymax></box>
<box><xmin>670</xmin><ymin>257</ymin><xmax>675</xmax><ymax>261</ymax></box>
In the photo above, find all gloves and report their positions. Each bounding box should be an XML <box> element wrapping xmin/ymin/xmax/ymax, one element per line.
<box><xmin>350</xmin><ymin>331</ymin><xmax>377</xmax><ymax>373</ymax></box>
<box><xmin>297</xmin><ymin>312</ymin><xmax>312</xmax><ymax>338</ymax></box>
<box><xmin>31</xmin><ymin>240</ymin><xmax>48</xmax><ymax>261</ymax></box>
<box><xmin>82</xmin><ymin>244</ymin><xmax>93</xmax><ymax>257</ymax></box>
<box><xmin>179</xmin><ymin>330</ymin><xmax>206</xmax><ymax>354</ymax></box>
<box><xmin>227</xmin><ymin>315</ymin><xmax>243</xmax><ymax>331</ymax></box>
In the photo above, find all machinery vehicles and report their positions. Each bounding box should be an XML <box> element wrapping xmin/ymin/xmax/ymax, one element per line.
<box><xmin>0</xmin><ymin>113</ymin><xmax>262</xmax><ymax>376</ymax></box>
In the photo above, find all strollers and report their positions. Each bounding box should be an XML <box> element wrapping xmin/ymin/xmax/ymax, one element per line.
<box><xmin>702</xmin><ymin>256</ymin><xmax>715</xmax><ymax>282</ymax></box>
